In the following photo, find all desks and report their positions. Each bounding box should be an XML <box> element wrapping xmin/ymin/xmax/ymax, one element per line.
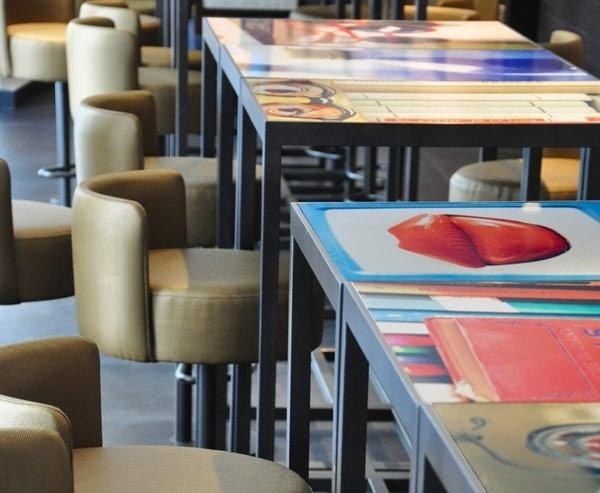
<box><xmin>196</xmin><ymin>13</ymin><xmax>600</xmax><ymax>462</ymax></box>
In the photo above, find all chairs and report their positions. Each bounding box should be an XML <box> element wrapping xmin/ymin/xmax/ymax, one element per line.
<box><xmin>444</xmin><ymin>147</ymin><xmax>585</xmax><ymax>205</ymax></box>
<box><xmin>400</xmin><ymin>0</ymin><xmax>510</xmax><ymax>25</ymax></box>
<box><xmin>0</xmin><ymin>156</ymin><xmax>78</xmax><ymax>307</ymax></box>
<box><xmin>1</xmin><ymin>0</ymin><xmax>68</xmax><ymax>91</ymax></box>
<box><xmin>66</xmin><ymin>85</ymin><xmax>268</xmax><ymax>250</ymax></box>
<box><xmin>61</xmin><ymin>11</ymin><xmax>211</xmax><ymax>153</ymax></box>
<box><xmin>76</xmin><ymin>0</ymin><xmax>205</xmax><ymax>78</ymax></box>
<box><xmin>1</xmin><ymin>335</ymin><xmax>315</xmax><ymax>492</ymax></box>
<box><xmin>72</xmin><ymin>168</ymin><xmax>326</xmax><ymax>454</ymax></box>
<box><xmin>533</xmin><ymin>27</ymin><xmax>590</xmax><ymax>160</ymax></box>
